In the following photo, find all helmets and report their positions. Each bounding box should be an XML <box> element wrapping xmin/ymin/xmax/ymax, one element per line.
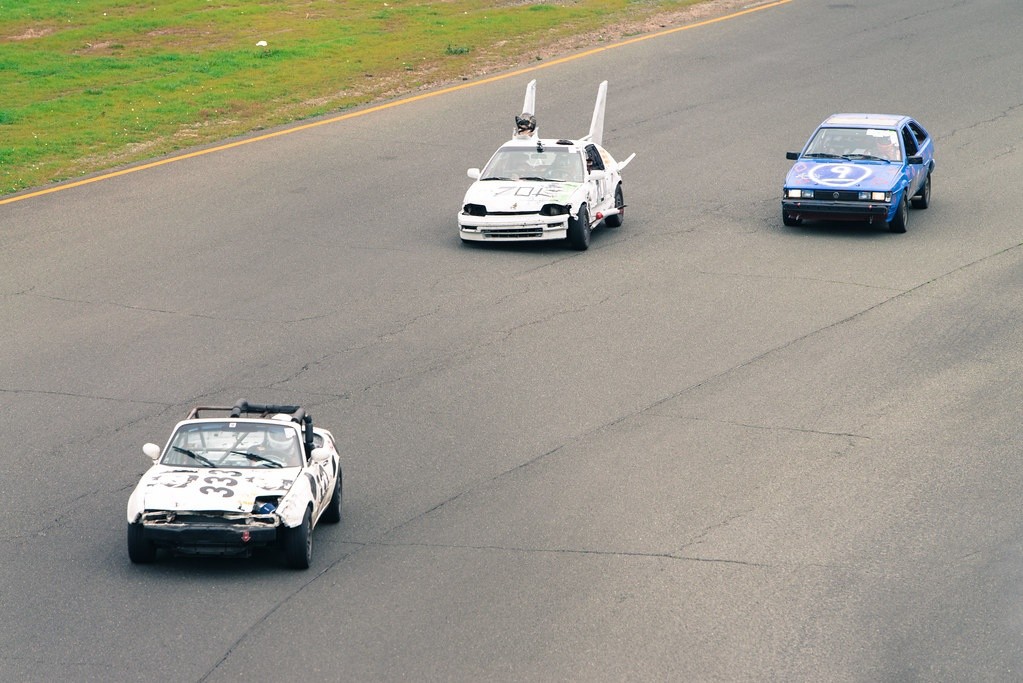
<box><xmin>873</xmin><ymin>133</ymin><xmax>894</xmax><ymax>153</ymax></box>
<box><xmin>267</xmin><ymin>414</ymin><xmax>296</xmax><ymax>451</ymax></box>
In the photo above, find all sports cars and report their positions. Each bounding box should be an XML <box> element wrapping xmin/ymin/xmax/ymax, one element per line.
<box><xmin>125</xmin><ymin>399</ymin><xmax>344</xmax><ymax>570</ymax></box>
<box><xmin>782</xmin><ymin>113</ymin><xmax>935</xmax><ymax>234</ymax></box>
<box><xmin>457</xmin><ymin>77</ymin><xmax>638</xmax><ymax>250</ymax></box>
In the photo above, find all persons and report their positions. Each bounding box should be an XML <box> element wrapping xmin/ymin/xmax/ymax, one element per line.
<box><xmin>865</xmin><ymin>136</ymin><xmax>900</xmax><ymax>161</ymax></box>
<box><xmin>543</xmin><ymin>154</ymin><xmax>580</xmax><ymax>182</ymax></box>
<box><xmin>247</xmin><ymin>414</ymin><xmax>303</xmax><ymax>467</ymax></box>
